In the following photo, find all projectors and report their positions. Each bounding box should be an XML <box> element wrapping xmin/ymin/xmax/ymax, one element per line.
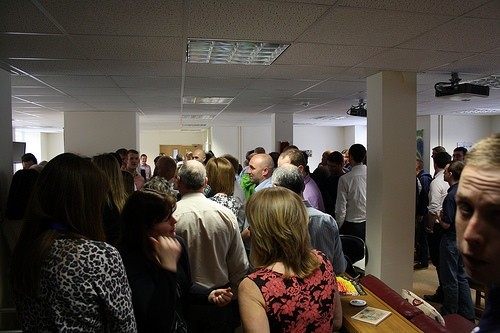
<box><xmin>435</xmin><ymin>83</ymin><xmax>490</xmax><ymax>101</ymax></box>
<box><xmin>350</xmin><ymin>109</ymin><xmax>367</xmax><ymax>117</ymax></box>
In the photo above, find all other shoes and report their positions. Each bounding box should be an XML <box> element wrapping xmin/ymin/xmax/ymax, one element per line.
<box><xmin>413</xmin><ymin>263</ymin><xmax>429</xmax><ymax>270</ymax></box>
<box><xmin>424</xmin><ymin>286</ymin><xmax>442</xmax><ymax>303</ymax></box>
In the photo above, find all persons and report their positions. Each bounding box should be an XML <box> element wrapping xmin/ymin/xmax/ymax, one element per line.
<box><xmin>8</xmin><ymin>153</ymin><xmax>138</xmax><ymax>333</ymax></box>
<box><xmin>141</xmin><ymin>156</ymin><xmax>179</xmax><ymax>200</ymax></box>
<box><xmin>456</xmin><ymin>134</ymin><xmax>500</xmax><ymax>333</ymax></box>
<box><xmin>424</xmin><ymin>152</ymin><xmax>452</xmax><ymax>303</ymax></box>
<box><xmin>335</xmin><ymin>144</ymin><xmax>367</xmax><ymax>278</ymax></box>
<box><xmin>238</xmin><ymin>186</ymin><xmax>342</xmax><ymax>333</ymax></box>
<box><xmin>240</xmin><ymin>153</ymin><xmax>275</xmax><ymax>257</ymax></box>
<box><xmin>140</xmin><ymin>157</ymin><xmax>177</xmax><ymax>196</ymax></box>
<box><xmin>413</xmin><ymin>160</ymin><xmax>431</xmax><ymax>271</ymax></box>
<box><xmin>434</xmin><ymin>161</ymin><xmax>476</xmax><ymax>325</ymax></box>
<box><xmin>113</xmin><ymin>189</ymin><xmax>233</xmax><ymax>333</ymax></box>
<box><xmin>205</xmin><ymin>156</ymin><xmax>248</xmax><ymax>237</ymax></box>
<box><xmin>1</xmin><ymin>170</ymin><xmax>40</xmax><ymax>255</ymax></box>
<box><xmin>452</xmin><ymin>148</ymin><xmax>468</xmax><ymax>161</ymax></box>
<box><xmin>433</xmin><ymin>147</ymin><xmax>445</xmax><ymax>175</ymax></box>
<box><xmin>277</xmin><ymin>149</ymin><xmax>325</xmax><ymax>212</ymax></box>
<box><xmin>20</xmin><ymin>141</ymin><xmax>467</xmax><ymax>186</ymax></box>
<box><xmin>249</xmin><ymin>164</ymin><xmax>347</xmax><ymax>276</ymax></box>
<box><xmin>172</xmin><ymin>160</ymin><xmax>248</xmax><ymax>286</ymax></box>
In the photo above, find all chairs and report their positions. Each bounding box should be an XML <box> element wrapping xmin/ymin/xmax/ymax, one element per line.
<box><xmin>339</xmin><ymin>235</ymin><xmax>366</xmax><ymax>278</ymax></box>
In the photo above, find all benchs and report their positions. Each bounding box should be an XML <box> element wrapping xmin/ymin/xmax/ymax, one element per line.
<box><xmin>359</xmin><ymin>274</ymin><xmax>476</xmax><ymax>333</ymax></box>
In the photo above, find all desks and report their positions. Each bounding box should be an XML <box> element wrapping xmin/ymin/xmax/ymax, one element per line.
<box><xmin>330</xmin><ymin>273</ymin><xmax>424</xmax><ymax>333</ymax></box>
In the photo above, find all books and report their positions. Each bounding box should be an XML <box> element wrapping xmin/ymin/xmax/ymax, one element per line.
<box><xmin>351</xmin><ymin>306</ymin><xmax>392</xmax><ymax>325</ymax></box>
